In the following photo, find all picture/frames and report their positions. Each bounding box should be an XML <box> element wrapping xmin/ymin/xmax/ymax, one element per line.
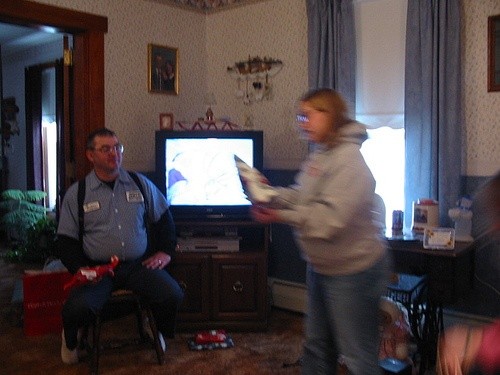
<box><xmin>487</xmin><ymin>14</ymin><xmax>500</xmax><ymax>93</ymax></box>
<box><xmin>147</xmin><ymin>43</ymin><xmax>179</xmax><ymax>97</ymax></box>
<box><xmin>423</xmin><ymin>228</ymin><xmax>454</xmax><ymax>248</ymax></box>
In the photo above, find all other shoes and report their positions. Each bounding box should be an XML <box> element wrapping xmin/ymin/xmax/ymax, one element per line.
<box><xmin>143</xmin><ymin>318</ymin><xmax>165</xmax><ymax>352</ymax></box>
<box><xmin>61</xmin><ymin>327</ymin><xmax>78</xmax><ymax>364</ymax></box>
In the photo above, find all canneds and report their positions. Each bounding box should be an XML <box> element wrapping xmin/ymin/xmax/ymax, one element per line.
<box><xmin>391</xmin><ymin>210</ymin><xmax>404</xmax><ymax>230</ymax></box>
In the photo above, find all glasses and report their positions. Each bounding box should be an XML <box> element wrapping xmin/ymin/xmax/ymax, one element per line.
<box><xmin>92</xmin><ymin>143</ymin><xmax>124</xmax><ymax>153</ymax></box>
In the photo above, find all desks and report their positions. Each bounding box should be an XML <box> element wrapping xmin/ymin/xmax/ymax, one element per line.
<box><xmin>375</xmin><ymin>238</ymin><xmax>474</xmax><ymax>375</ymax></box>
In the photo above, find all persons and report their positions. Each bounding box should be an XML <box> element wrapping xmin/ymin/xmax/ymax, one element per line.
<box><xmin>58</xmin><ymin>128</ymin><xmax>186</xmax><ymax>365</ymax></box>
<box><xmin>243</xmin><ymin>89</ymin><xmax>395</xmax><ymax>375</ymax></box>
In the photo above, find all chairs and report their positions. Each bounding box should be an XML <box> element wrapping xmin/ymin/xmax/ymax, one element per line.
<box><xmin>80</xmin><ymin>289</ymin><xmax>165</xmax><ymax>374</ymax></box>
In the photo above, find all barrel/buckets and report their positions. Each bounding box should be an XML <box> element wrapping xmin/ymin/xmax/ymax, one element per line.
<box><xmin>412</xmin><ymin>201</ymin><xmax>439</xmax><ymax>233</ymax></box>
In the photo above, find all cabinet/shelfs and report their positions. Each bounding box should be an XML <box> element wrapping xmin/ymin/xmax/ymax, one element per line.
<box><xmin>157</xmin><ymin>211</ymin><xmax>272</xmax><ymax>334</ymax></box>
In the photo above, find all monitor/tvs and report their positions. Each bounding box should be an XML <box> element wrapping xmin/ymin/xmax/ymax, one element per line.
<box><xmin>153</xmin><ymin>130</ymin><xmax>266</xmax><ymax>219</ymax></box>
